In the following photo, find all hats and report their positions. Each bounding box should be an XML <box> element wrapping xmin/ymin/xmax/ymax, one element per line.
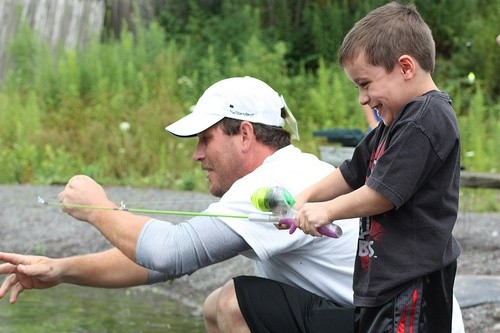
<box><xmin>165</xmin><ymin>75</ymin><xmax>300</xmax><ymax>140</ymax></box>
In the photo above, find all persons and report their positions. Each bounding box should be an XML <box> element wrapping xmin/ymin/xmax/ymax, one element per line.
<box><xmin>0</xmin><ymin>77</ymin><xmax>464</xmax><ymax>333</ymax></box>
<box><xmin>364</xmin><ymin>104</ymin><xmax>383</xmax><ymax>136</ymax></box>
<box><xmin>269</xmin><ymin>4</ymin><xmax>460</xmax><ymax>333</ymax></box>
